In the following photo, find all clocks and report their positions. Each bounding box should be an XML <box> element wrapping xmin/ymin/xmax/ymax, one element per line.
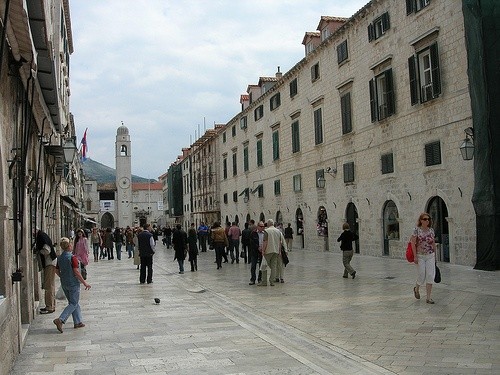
<box><xmin>119</xmin><ymin>177</ymin><xmax>130</xmax><ymax>189</ymax></box>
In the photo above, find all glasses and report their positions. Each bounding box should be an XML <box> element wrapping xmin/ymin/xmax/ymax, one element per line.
<box><xmin>421</xmin><ymin>217</ymin><xmax>431</xmax><ymax>222</ymax></box>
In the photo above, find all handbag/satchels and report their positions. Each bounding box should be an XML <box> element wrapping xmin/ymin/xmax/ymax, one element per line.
<box><xmin>56</xmin><ymin>284</ymin><xmax>67</xmax><ymax>300</ymax></box>
<box><xmin>407</xmin><ymin>233</ymin><xmax>417</xmax><ymax>263</ymax></box>
<box><xmin>434</xmin><ymin>261</ymin><xmax>441</xmax><ymax>283</ymax></box>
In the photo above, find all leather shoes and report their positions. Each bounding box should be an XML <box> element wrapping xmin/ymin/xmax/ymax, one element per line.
<box><xmin>342</xmin><ymin>275</ymin><xmax>348</xmax><ymax>278</ymax></box>
<box><xmin>40</xmin><ymin>309</ymin><xmax>53</xmax><ymax>314</ymax></box>
<box><xmin>40</xmin><ymin>308</ymin><xmax>55</xmax><ymax>312</ymax></box>
<box><xmin>352</xmin><ymin>271</ymin><xmax>356</xmax><ymax>279</ymax></box>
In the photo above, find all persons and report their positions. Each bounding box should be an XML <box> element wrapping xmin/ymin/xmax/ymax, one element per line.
<box><xmin>52</xmin><ymin>238</ymin><xmax>92</xmax><ymax>333</ymax></box>
<box><xmin>33</xmin><ymin>229</ymin><xmax>57</xmax><ymax>314</ymax></box>
<box><xmin>94</xmin><ymin>219</ymin><xmax>295</xmax><ymax>286</ymax></box>
<box><xmin>411</xmin><ymin>213</ymin><xmax>436</xmax><ymax>305</ymax></box>
<box><xmin>335</xmin><ymin>224</ymin><xmax>358</xmax><ymax>280</ymax></box>
<box><xmin>72</xmin><ymin>229</ymin><xmax>90</xmax><ymax>280</ymax></box>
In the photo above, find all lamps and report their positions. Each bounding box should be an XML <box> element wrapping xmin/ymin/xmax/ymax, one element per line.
<box><xmin>317</xmin><ymin>167</ymin><xmax>337</xmax><ymax>188</ymax></box>
<box><xmin>62</xmin><ymin>138</ymin><xmax>77</xmax><ymax>164</ymax></box>
<box><xmin>243</xmin><ymin>195</ymin><xmax>249</xmax><ymax>203</ymax></box>
<box><xmin>459</xmin><ymin>127</ymin><xmax>475</xmax><ymax>160</ymax></box>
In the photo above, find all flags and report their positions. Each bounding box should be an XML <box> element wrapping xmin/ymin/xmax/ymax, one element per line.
<box><xmin>81</xmin><ymin>132</ymin><xmax>90</xmax><ymax>161</ymax></box>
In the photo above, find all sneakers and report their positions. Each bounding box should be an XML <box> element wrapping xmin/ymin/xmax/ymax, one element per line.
<box><xmin>414</xmin><ymin>286</ymin><xmax>420</xmax><ymax>299</ymax></box>
<box><xmin>74</xmin><ymin>323</ymin><xmax>86</xmax><ymax>328</ymax></box>
<box><xmin>426</xmin><ymin>298</ymin><xmax>435</xmax><ymax>304</ymax></box>
<box><xmin>73</xmin><ymin>239</ymin><xmax>304</xmax><ymax>292</ymax></box>
<box><xmin>53</xmin><ymin>319</ymin><xmax>63</xmax><ymax>333</ymax></box>
